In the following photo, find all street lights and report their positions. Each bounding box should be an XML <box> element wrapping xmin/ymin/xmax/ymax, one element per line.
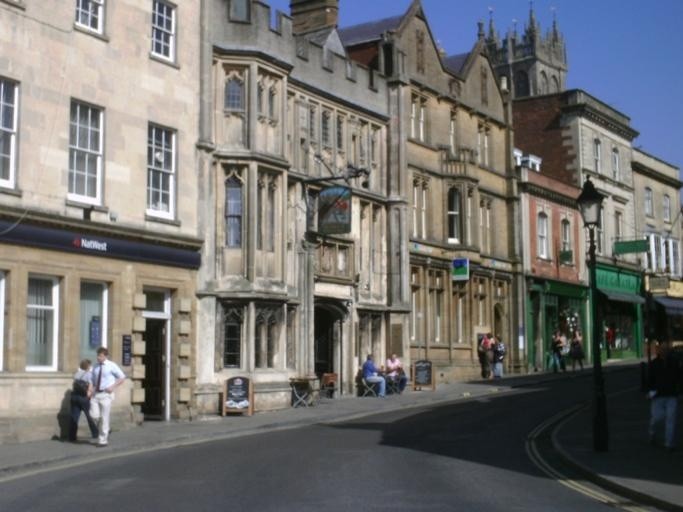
<box><xmin>571</xmin><ymin>172</ymin><xmax>614</xmax><ymax>458</ymax></box>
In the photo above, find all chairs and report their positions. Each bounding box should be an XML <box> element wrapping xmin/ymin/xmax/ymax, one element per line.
<box><xmin>320</xmin><ymin>373</ymin><xmax>338</xmax><ymax>391</ymax></box>
<box><xmin>359</xmin><ymin>366</ymin><xmax>403</xmax><ymax>397</ymax></box>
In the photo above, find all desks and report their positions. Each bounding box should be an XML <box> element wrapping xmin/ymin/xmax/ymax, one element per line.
<box><xmin>290</xmin><ymin>376</ymin><xmax>317</xmax><ymax>407</ymax></box>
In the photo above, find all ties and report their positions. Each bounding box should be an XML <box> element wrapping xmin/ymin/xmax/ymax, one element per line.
<box><xmin>94</xmin><ymin>363</ymin><xmax>102</xmax><ymax>392</ymax></box>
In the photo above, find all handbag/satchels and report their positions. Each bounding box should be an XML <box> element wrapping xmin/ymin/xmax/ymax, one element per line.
<box><xmin>71</xmin><ymin>378</ymin><xmax>90</xmax><ymax>397</ymax></box>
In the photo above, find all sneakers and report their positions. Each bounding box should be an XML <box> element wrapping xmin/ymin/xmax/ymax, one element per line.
<box><xmin>88</xmin><ymin>428</ymin><xmax>112</xmax><ymax>447</ymax></box>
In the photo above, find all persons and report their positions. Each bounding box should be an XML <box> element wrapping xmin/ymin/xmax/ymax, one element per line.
<box><xmin>644</xmin><ymin>344</ymin><xmax>683</xmax><ymax>452</ymax></box>
<box><xmin>68</xmin><ymin>359</ymin><xmax>100</xmax><ymax>442</ymax></box>
<box><xmin>87</xmin><ymin>347</ymin><xmax>126</xmax><ymax>447</ymax></box>
<box><xmin>361</xmin><ymin>354</ymin><xmax>386</xmax><ymax>399</ymax></box>
<box><xmin>477</xmin><ymin>333</ymin><xmax>506</xmax><ymax>386</ymax></box>
<box><xmin>384</xmin><ymin>353</ymin><xmax>408</xmax><ymax>393</ymax></box>
<box><xmin>551</xmin><ymin>329</ymin><xmax>568</xmax><ymax>374</ymax></box>
<box><xmin>569</xmin><ymin>330</ymin><xmax>586</xmax><ymax>371</ymax></box>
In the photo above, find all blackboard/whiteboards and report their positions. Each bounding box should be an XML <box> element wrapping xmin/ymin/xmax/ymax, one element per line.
<box><xmin>222</xmin><ymin>377</ymin><xmax>252</xmax><ymax>412</ymax></box>
<box><xmin>412</xmin><ymin>360</ymin><xmax>434</xmax><ymax>388</ymax></box>
